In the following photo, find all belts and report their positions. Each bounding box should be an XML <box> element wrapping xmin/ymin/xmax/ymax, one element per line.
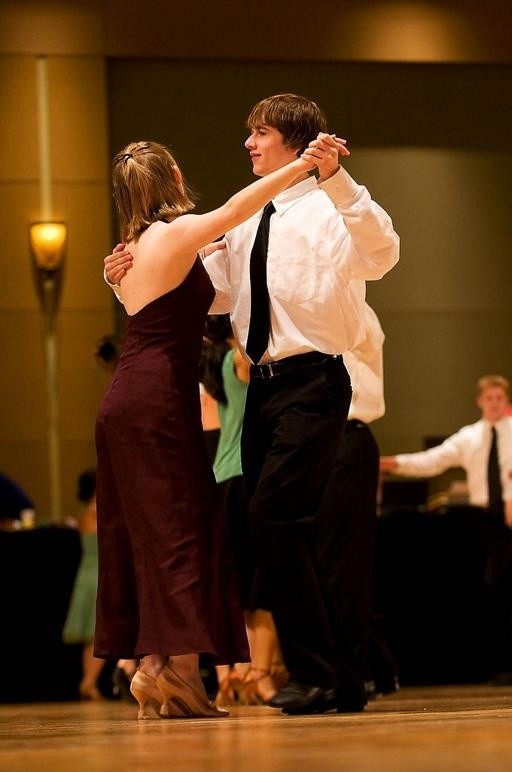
<box><xmin>248</xmin><ymin>352</ymin><xmax>319</xmax><ymax>378</ymax></box>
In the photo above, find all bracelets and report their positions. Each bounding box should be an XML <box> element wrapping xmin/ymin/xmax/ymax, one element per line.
<box><xmin>199</xmin><ymin>245</ymin><xmax>205</xmax><ymax>260</ymax></box>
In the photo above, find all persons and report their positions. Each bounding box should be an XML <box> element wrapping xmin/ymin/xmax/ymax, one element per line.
<box><xmin>61</xmin><ymin>466</ymin><xmax>113</xmax><ymax>700</ymax></box>
<box><xmin>103</xmin><ymin>93</ymin><xmax>401</xmax><ymax>715</ymax></box>
<box><xmin>379</xmin><ymin>371</ymin><xmax>511</xmax><ymax>688</ymax></box>
<box><xmin>203</xmin><ymin>301</ymin><xmax>280</xmax><ymax>707</ymax></box>
<box><xmin>90</xmin><ymin>131</ymin><xmax>349</xmax><ymax>718</ymax></box>
<box><xmin>1</xmin><ymin>470</ymin><xmax>36</xmax><ymax>531</ymax></box>
<box><xmin>189</xmin><ymin>377</ymin><xmax>249</xmax><ymax>711</ymax></box>
<box><xmin>333</xmin><ymin>295</ymin><xmax>398</xmax><ymax>701</ymax></box>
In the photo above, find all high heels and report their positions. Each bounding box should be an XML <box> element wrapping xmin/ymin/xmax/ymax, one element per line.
<box><xmin>156</xmin><ymin>666</ymin><xmax>228</xmax><ymax>719</ymax></box>
<box><xmin>131</xmin><ymin>669</ymin><xmax>162</xmax><ymax>717</ymax></box>
<box><xmin>213</xmin><ymin>660</ymin><xmax>290</xmax><ymax>708</ymax></box>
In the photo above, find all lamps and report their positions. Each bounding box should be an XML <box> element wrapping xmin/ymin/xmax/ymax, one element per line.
<box><xmin>28</xmin><ymin>220</ymin><xmax>70</xmax><ymax>329</ymax></box>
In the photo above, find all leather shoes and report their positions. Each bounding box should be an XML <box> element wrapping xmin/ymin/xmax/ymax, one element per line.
<box><xmin>113</xmin><ymin>667</ymin><xmax>136</xmax><ymax>706</ymax></box>
<box><xmin>268</xmin><ymin>679</ymin><xmax>364</xmax><ymax>715</ymax></box>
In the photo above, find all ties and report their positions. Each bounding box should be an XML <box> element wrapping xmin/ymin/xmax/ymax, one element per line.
<box><xmin>486</xmin><ymin>428</ymin><xmax>502</xmax><ymax>508</ymax></box>
<box><xmin>245</xmin><ymin>202</ymin><xmax>276</xmax><ymax>365</ymax></box>
